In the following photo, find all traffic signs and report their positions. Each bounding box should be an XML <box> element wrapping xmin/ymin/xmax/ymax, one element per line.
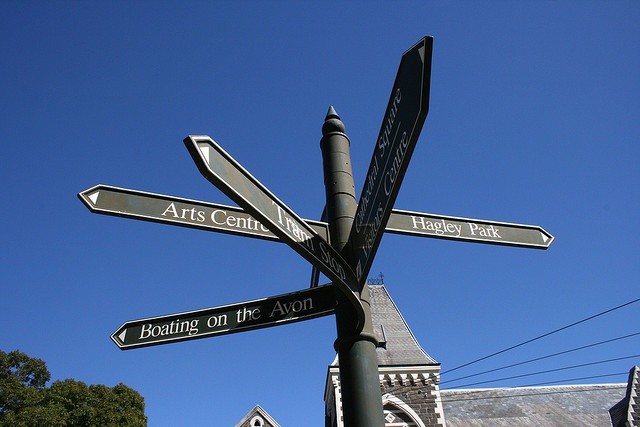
<box><xmin>78</xmin><ymin>183</ymin><xmax>331</xmax><ymax>246</ymax></box>
<box><xmin>183</xmin><ymin>135</ymin><xmax>365</xmax><ymax>334</ymax></box>
<box><xmin>346</xmin><ymin>36</ymin><xmax>434</xmax><ymax>293</ymax></box>
<box><xmin>384</xmin><ymin>209</ymin><xmax>555</xmax><ymax>250</ymax></box>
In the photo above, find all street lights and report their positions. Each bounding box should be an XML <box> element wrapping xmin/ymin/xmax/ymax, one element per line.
<box><xmin>110</xmin><ymin>282</ymin><xmax>335</xmax><ymax>350</ymax></box>
